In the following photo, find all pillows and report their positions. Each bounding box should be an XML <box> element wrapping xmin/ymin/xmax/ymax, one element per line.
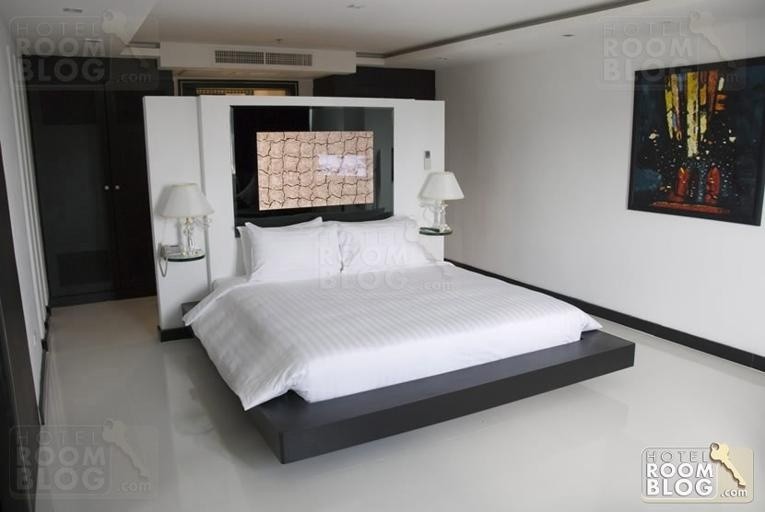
<box><xmin>235</xmin><ymin>213</ymin><xmax>429</xmax><ymax>288</ymax></box>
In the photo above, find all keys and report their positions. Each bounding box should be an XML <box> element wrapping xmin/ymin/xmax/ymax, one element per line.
<box><xmin>710</xmin><ymin>441</ymin><xmax>747</xmax><ymax>486</ymax></box>
<box><xmin>102</xmin><ymin>418</ymin><xmax>149</xmax><ymax>478</ymax></box>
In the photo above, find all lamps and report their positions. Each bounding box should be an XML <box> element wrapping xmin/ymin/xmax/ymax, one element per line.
<box><xmin>158</xmin><ymin>183</ymin><xmax>216</xmax><ymax>257</ymax></box>
<box><xmin>418</xmin><ymin>170</ymin><xmax>466</xmax><ymax>231</ymax></box>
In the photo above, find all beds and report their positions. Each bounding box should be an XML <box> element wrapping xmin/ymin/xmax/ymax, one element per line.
<box><xmin>178</xmin><ymin>258</ymin><xmax>639</xmax><ymax>467</ymax></box>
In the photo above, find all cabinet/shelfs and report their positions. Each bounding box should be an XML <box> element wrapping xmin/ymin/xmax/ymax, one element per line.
<box><xmin>23</xmin><ymin>65</ymin><xmax>172</xmax><ymax>311</ymax></box>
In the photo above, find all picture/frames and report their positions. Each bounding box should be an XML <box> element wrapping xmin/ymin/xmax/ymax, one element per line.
<box><xmin>625</xmin><ymin>56</ymin><xmax>764</xmax><ymax>227</ymax></box>
<box><xmin>176</xmin><ymin>78</ymin><xmax>300</xmax><ymax>96</ymax></box>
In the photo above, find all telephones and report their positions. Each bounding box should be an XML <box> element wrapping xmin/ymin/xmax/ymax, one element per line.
<box><xmin>161</xmin><ymin>245</ymin><xmax>183</xmax><ymax>258</ymax></box>
